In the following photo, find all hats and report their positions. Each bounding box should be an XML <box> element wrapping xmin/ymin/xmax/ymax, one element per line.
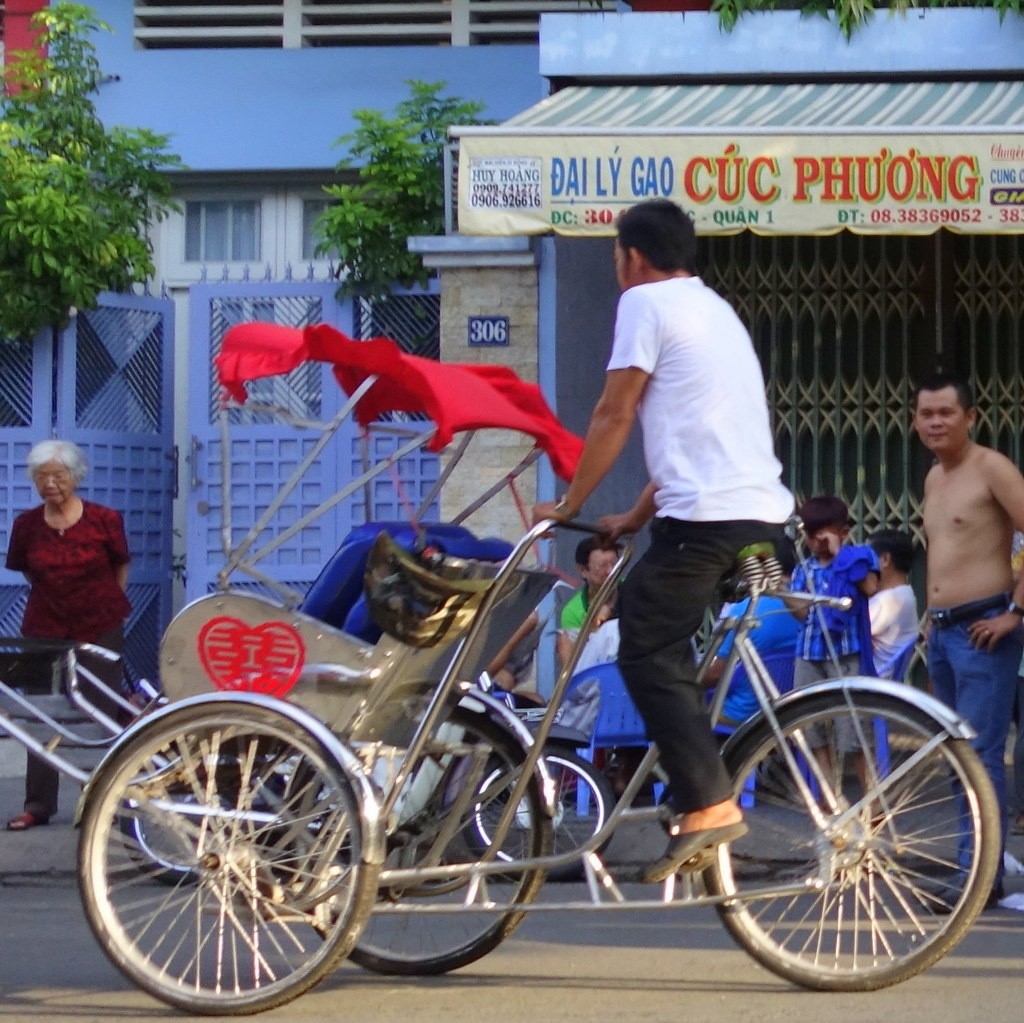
<box><xmin>800</xmin><ymin>494</ymin><xmax>847</xmax><ymax>532</ymax></box>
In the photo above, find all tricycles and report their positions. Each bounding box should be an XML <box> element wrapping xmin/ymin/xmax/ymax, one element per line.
<box><xmin>72</xmin><ymin>322</ymin><xmax>1004</xmax><ymax>1018</ymax></box>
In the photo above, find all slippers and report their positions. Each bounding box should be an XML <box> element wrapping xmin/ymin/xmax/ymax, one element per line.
<box><xmin>638</xmin><ymin>821</ymin><xmax>748</xmax><ymax>882</ymax></box>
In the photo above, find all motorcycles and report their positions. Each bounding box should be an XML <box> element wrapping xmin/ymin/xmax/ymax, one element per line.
<box><xmin>125</xmin><ymin>667</ymin><xmax>620</xmax><ymax>892</ymax></box>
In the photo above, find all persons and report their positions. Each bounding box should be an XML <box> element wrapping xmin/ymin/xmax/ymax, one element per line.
<box><xmin>533</xmin><ymin>197</ymin><xmax>796</xmax><ymax>883</ymax></box>
<box><xmin>5</xmin><ymin>441</ymin><xmax>134</xmax><ymax>830</ymax></box>
<box><xmin>916</xmin><ymin>370</ymin><xmax>1024</xmax><ymax>912</ymax></box>
<box><xmin>560</xmin><ymin>494</ymin><xmax>919</xmax><ymax>800</ymax></box>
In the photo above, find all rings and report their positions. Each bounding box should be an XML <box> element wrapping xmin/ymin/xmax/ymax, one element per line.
<box><xmin>985</xmin><ymin>630</ymin><xmax>989</xmax><ymax>635</ymax></box>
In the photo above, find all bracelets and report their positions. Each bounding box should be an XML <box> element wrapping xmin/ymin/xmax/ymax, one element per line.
<box><xmin>553</xmin><ymin>494</ymin><xmax>580</xmax><ymax>518</ymax></box>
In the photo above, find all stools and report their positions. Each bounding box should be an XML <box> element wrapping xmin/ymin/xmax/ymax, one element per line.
<box><xmin>716</xmin><ymin>725</ymin><xmax>820</xmax><ymax>809</ymax></box>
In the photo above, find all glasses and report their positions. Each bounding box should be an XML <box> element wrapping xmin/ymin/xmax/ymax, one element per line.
<box><xmin>584</xmin><ymin>558</ymin><xmax>618</xmax><ymax>570</ymax></box>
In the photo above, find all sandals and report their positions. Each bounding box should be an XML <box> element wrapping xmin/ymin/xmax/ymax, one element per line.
<box><xmin>74</xmin><ymin>815</ymin><xmax>118</xmax><ymax>829</ymax></box>
<box><xmin>7</xmin><ymin>812</ymin><xmax>49</xmax><ymax>830</ymax></box>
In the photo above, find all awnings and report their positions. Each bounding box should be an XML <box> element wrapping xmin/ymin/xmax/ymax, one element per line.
<box><xmin>446</xmin><ymin>82</ymin><xmax>1024</xmax><ymax>239</ymax></box>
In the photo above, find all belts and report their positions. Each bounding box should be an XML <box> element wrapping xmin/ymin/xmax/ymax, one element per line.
<box><xmin>929</xmin><ymin>597</ymin><xmax>1013</xmax><ymax>628</ymax></box>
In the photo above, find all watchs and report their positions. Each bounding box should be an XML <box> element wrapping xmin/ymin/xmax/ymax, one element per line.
<box><xmin>1007</xmin><ymin>601</ymin><xmax>1024</xmax><ymax>616</ymax></box>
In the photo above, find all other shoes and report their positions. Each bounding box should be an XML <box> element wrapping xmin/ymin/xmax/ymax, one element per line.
<box><xmin>922</xmin><ymin>887</ymin><xmax>998</xmax><ymax>915</ymax></box>
<box><xmin>929</xmin><ymin>878</ymin><xmax>1004</xmax><ymax>901</ymax></box>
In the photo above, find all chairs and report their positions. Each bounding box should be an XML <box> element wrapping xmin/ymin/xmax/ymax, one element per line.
<box><xmin>297</xmin><ymin>521</ymin><xmax>516</xmax><ymax>643</ymax></box>
<box><xmin>791</xmin><ymin>635</ymin><xmax>923</xmax><ymax>794</ymax></box>
<box><xmin>557</xmin><ymin>663</ymin><xmax>669</xmax><ymax>815</ymax></box>
